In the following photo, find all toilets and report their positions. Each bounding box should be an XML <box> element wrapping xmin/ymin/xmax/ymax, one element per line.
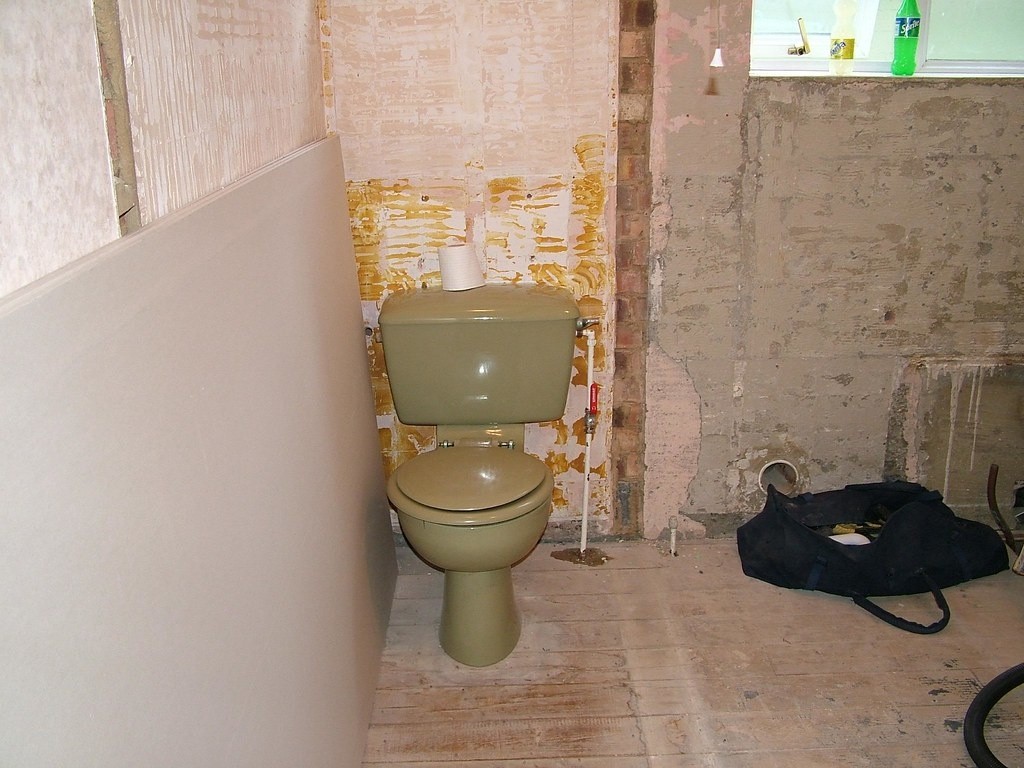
<box><xmin>374</xmin><ymin>280</ymin><xmax>586</xmax><ymax>670</ymax></box>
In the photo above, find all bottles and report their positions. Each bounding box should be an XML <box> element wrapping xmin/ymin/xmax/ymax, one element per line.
<box><xmin>830</xmin><ymin>0</ymin><xmax>855</xmax><ymax>72</ymax></box>
<box><xmin>892</xmin><ymin>0</ymin><xmax>921</xmax><ymax>77</ymax></box>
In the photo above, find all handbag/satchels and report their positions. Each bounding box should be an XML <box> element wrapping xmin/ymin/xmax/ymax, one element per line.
<box><xmin>734</xmin><ymin>481</ymin><xmax>1012</xmax><ymax>635</ymax></box>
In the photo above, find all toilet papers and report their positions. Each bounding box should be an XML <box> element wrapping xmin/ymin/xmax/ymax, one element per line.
<box><xmin>434</xmin><ymin>238</ymin><xmax>483</xmax><ymax>295</ymax></box>
